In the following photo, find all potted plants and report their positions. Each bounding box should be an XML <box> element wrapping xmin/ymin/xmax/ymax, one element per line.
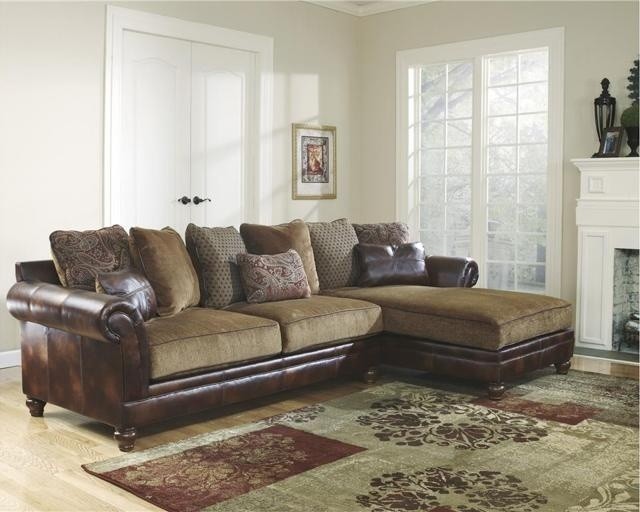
<box><xmin>620</xmin><ymin>107</ymin><xmax>639</xmax><ymax>157</ymax></box>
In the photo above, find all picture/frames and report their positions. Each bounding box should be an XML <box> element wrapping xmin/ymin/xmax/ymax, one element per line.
<box><xmin>599</xmin><ymin>127</ymin><xmax>623</xmax><ymax>158</ymax></box>
<box><xmin>292</xmin><ymin>123</ymin><xmax>336</xmax><ymax>200</ymax></box>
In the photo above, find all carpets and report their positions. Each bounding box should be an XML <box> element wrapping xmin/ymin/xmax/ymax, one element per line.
<box><xmin>80</xmin><ymin>365</ymin><xmax>639</xmax><ymax>511</ymax></box>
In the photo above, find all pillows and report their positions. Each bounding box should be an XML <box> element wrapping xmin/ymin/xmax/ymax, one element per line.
<box><xmin>48</xmin><ymin>225</ymin><xmax>135</xmax><ymax>292</ymax></box>
<box><xmin>353</xmin><ymin>241</ymin><xmax>430</xmax><ymax>286</ymax></box>
<box><xmin>129</xmin><ymin>225</ymin><xmax>200</xmax><ymax>319</ymax></box>
<box><xmin>235</xmin><ymin>248</ymin><xmax>311</xmax><ymax>303</ymax></box>
<box><xmin>305</xmin><ymin>218</ymin><xmax>360</xmax><ymax>289</ymax></box>
<box><xmin>240</xmin><ymin>219</ymin><xmax>321</xmax><ymax>294</ymax></box>
<box><xmin>185</xmin><ymin>222</ymin><xmax>250</xmax><ymax>308</ymax></box>
<box><xmin>95</xmin><ymin>266</ymin><xmax>157</xmax><ymax>322</ymax></box>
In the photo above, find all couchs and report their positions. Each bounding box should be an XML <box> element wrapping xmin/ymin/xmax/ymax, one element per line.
<box><xmin>6</xmin><ymin>219</ymin><xmax>576</xmax><ymax>452</ymax></box>
